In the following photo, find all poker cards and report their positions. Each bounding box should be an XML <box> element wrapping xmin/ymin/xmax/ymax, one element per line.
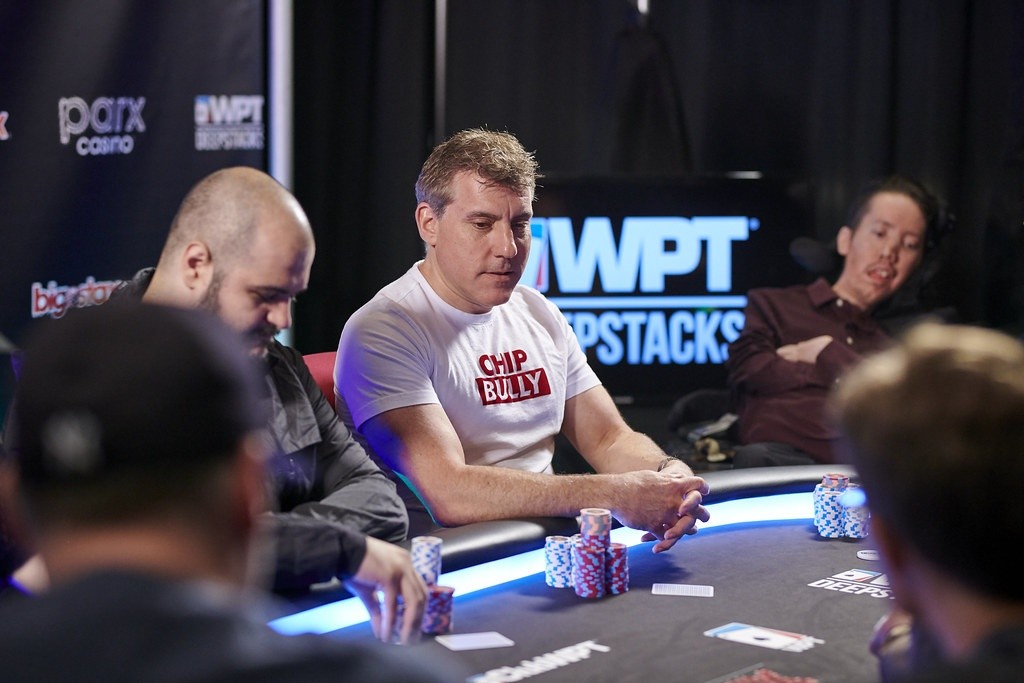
<box><xmin>832</xmin><ymin>568</ymin><xmax>882</xmax><ymax>582</ymax></box>
<box><xmin>434</xmin><ymin>631</ymin><xmax>516</xmax><ymax>652</ymax></box>
<box><xmin>651</xmin><ymin>582</ymin><xmax>714</xmax><ymax>598</ymax></box>
<box><xmin>869</xmin><ymin>573</ymin><xmax>890</xmax><ymax>586</ymax></box>
<box><xmin>702</xmin><ymin>620</ymin><xmax>807</xmax><ymax>650</ymax></box>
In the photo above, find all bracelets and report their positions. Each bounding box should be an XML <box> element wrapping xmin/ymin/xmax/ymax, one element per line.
<box><xmin>658</xmin><ymin>458</ymin><xmax>678</xmax><ymax>472</ymax></box>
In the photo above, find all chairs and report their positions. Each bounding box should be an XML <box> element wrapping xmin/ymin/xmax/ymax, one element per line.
<box><xmin>674</xmin><ymin>385</ymin><xmax>747</xmax><ymax>475</ymax></box>
<box><xmin>300</xmin><ymin>349</ymin><xmax>638</xmax><ymax>526</ymax></box>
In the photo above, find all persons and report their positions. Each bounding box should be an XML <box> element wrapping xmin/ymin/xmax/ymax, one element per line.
<box><xmin>332</xmin><ymin>129</ymin><xmax>711</xmax><ymax>555</ymax></box>
<box><xmin>725</xmin><ymin>174</ymin><xmax>931</xmax><ymax>472</ymax></box>
<box><xmin>106</xmin><ymin>166</ymin><xmax>431</xmax><ymax>647</ymax></box>
<box><xmin>830</xmin><ymin>323</ymin><xmax>1024</xmax><ymax>683</ymax></box>
<box><xmin>0</xmin><ymin>299</ymin><xmax>443</xmax><ymax>683</ymax></box>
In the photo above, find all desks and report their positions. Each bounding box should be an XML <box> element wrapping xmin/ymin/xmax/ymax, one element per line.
<box><xmin>256</xmin><ymin>465</ymin><xmax>896</xmax><ymax>683</ymax></box>
